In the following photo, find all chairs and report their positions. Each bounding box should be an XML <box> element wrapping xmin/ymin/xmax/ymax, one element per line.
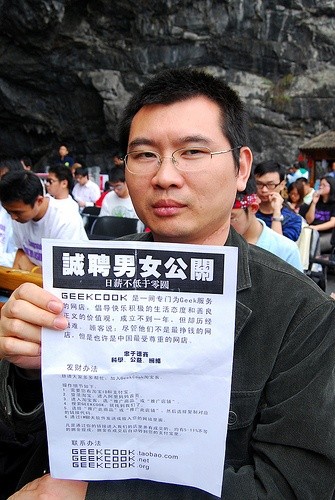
<box><xmin>298</xmin><ymin>230</ymin><xmax>335</xmax><ymax>293</ymax></box>
<box><xmin>80</xmin><ymin>205</ymin><xmax>141</xmax><ymax>241</ymax></box>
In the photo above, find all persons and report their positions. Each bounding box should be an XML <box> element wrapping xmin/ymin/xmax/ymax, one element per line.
<box><xmin>1</xmin><ymin>145</ymin><xmax>145</xmax><ymax>273</ymax></box>
<box><xmin>0</xmin><ymin>70</ymin><xmax>335</xmax><ymax>500</ymax></box>
<box><xmin>231</xmin><ymin>160</ymin><xmax>335</xmax><ymax>275</ymax></box>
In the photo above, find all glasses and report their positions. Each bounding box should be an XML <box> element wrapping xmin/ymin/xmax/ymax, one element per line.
<box><xmin>123</xmin><ymin>144</ymin><xmax>242</xmax><ymax>175</ymax></box>
<box><xmin>110</xmin><ymin>185</ymin><xmax>123</xmax><ymax>190</ymax></box>
<box><xmin>255</xmin><ymin>181</ymin><xmax>281</xmax><ymax>190</ymax></box>
<box><xmin>46</xmin><ymin>178</ymin><xmax>56</xmax><ymax>185</ymax></box>
<box><xmin>230</xmin><ymin>208</ymin><xmax>242</xmax><ymax>228</ymax></box>
